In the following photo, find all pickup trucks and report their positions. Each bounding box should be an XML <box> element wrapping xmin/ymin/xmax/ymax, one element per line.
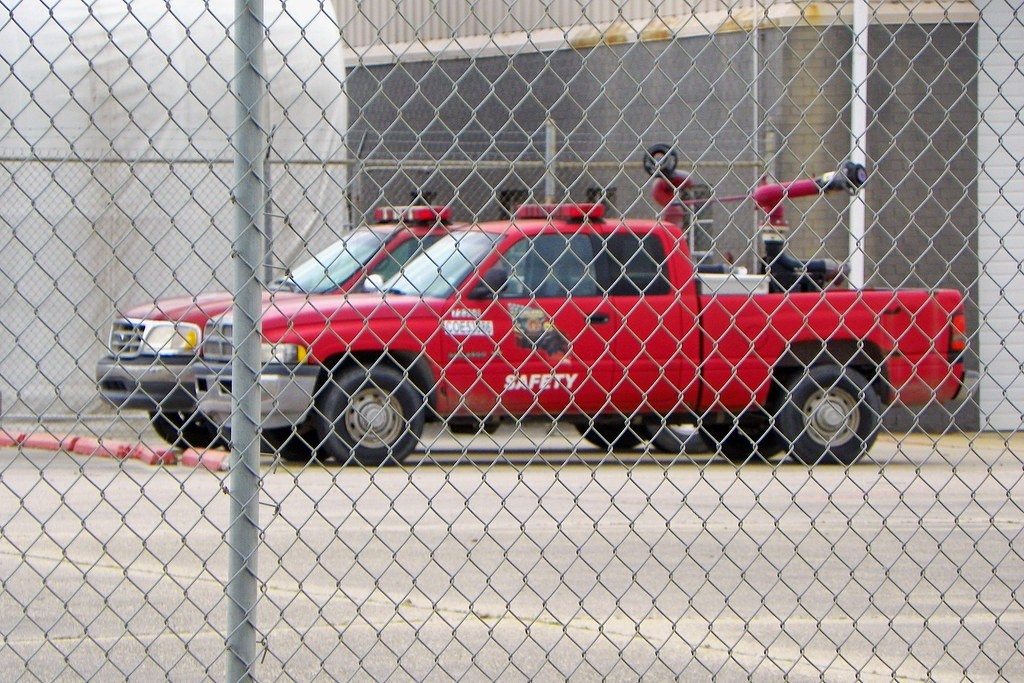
<box><xmin>194</xmin><ymin>160</ymin><xmax>964</xmax><ymax>474</ymax></box>
<box><xmin>95</xmin><ymin>199</ymin><xmax>470</xmax><ymax>452</ymax></box>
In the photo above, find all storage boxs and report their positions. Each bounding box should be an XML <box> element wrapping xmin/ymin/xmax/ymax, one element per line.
<box><xmin>695</xmin><ymin>271</ymin><xmax>770</xmax><ymax>293</ymax></box>
